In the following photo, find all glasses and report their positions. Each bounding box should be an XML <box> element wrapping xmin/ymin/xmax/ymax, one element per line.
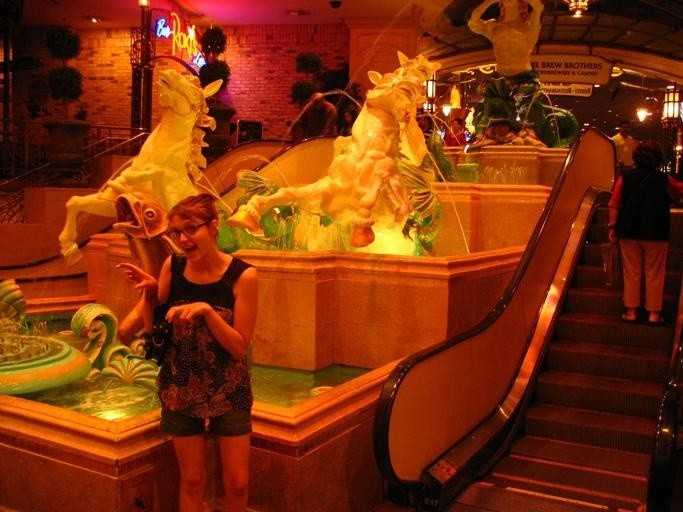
<box><xmin>170</xmin><ymin>223</ymin><xmax>209</xmax><ymax>239</ymax></box>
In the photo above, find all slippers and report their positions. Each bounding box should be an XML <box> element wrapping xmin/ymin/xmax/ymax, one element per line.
<box><xmin>622</xmin><ymin>313</ymin><xmax>664</xmax><ymax>326</ymax></box>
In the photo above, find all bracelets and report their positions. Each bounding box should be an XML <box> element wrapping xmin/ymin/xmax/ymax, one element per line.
<box><xmin>608</xmin><ymin>225</ymin><xmax>615</xmax><ymax>230</ymax></box>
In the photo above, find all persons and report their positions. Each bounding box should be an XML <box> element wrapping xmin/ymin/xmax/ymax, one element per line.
<box><xmin>607</xmin><ymin>139</ymin><xmax>683</xmax><ymax>327</ymax></box>
<box><xmin>115</xmin><ymin>192</ymin><xmax>259</xmax><ymax>512</ymax></box>
<box><xmin>286</xmin><ymin>54</ymin><xmax>469</xmax><ymax>147</ymax></box>
<box><xmin>468</xmin><ymin>0</ymin><xmax>552</xmax><ymax>148</ymax></box>
<box><xmin>608</xmin><ymin>119</ymin><xmax>639</xmax><ymax>178</ymax></box>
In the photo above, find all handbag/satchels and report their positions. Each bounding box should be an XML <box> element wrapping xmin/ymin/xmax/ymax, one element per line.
<box><xmin>600</xmin><ymin>242</ymin><xmax>622</xmax><ymax>286</ymax></box>
